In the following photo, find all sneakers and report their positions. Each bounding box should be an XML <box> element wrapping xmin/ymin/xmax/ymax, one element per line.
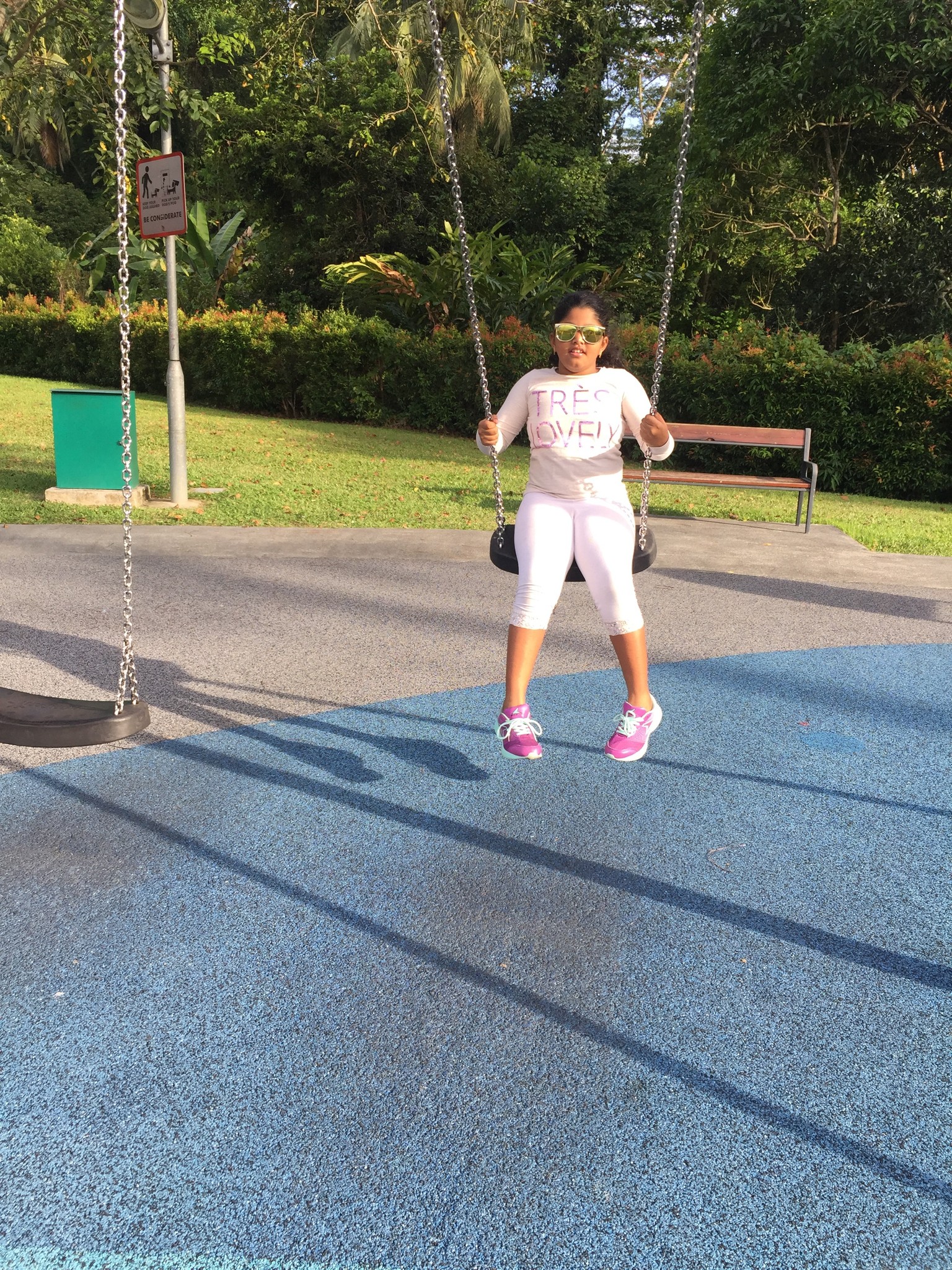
<box><xmin>603</xmin><ymin>692</ymin><xmax>662</xmax><ymax>762</ymax></box>
<box><xmin>494</xmin><ymin>702</ymin><xmax>543</xmax><ymax>759</ymax></box>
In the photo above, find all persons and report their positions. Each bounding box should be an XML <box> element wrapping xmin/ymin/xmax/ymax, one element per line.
<box><xmin>475</xmin><ymin>291</ymin><xmax>674</xmax><ymax>764</ymax></box>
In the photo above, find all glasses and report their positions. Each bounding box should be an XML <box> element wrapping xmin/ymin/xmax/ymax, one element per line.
<box><xmin>554</xmin><ymin>323</ymin><xmax>606</xmax><ymax>345</ymax></box>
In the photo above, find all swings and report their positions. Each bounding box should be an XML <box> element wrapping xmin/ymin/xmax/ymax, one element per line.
<box><xmin>0</xmin><ymin>0</ymin><xmax>153</xmax><ymax>750</ymax></box>
<box><xmin>423</xmin><ymin>3</ymin><xmax>705</xmax><ymax>578</ymax></box>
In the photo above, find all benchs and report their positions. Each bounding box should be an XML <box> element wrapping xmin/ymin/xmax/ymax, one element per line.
<box><xmin>623</xmin><ymin>421</ymin><xmax>819</xmax><ymax>535</ymax></box>
